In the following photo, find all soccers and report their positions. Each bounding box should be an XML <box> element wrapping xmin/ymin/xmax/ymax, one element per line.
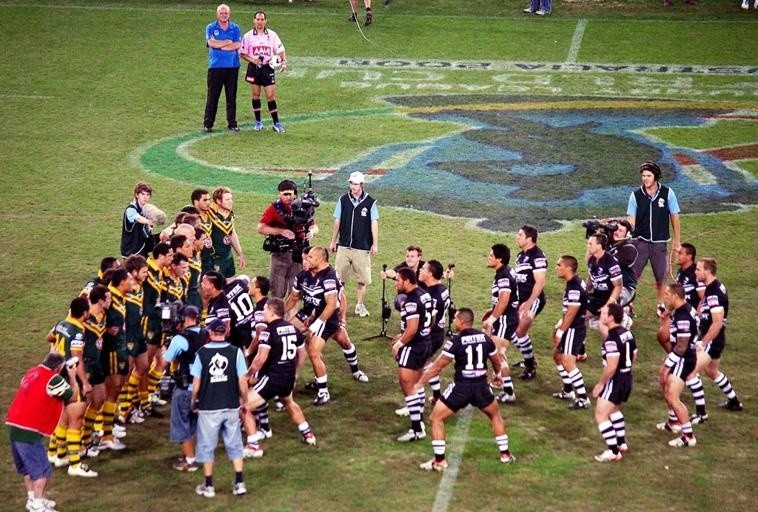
<box><xmin>269</xmin><ymin>55</ymin><xmax>282</xmax><ymax>69</ymax></box>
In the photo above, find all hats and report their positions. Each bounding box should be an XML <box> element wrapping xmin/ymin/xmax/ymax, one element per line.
<box><xmin>184</xmin><ymin>305</ymin><xmax>199</xmax><ymax>319</ymax></box>
<box><xmin>205</xmin><ymin>319</ymin><xmax>226</xmax><ymax>332</ymax></box>
<box><xmin>347</xmin><ymin>171</ymin><xmax>365</xmax><ymax>184</ymax></box>
<box><xmin>279</xmin><ymin>180</ymin><xmax>296</xmax><ymax>194</ymax></box>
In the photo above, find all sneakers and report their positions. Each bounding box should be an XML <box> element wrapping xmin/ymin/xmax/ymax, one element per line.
<box><xmin>112</xmin><ymin>427</ymin><xmax>127</xmax><ymax>438</ymax></box>
<box><xmin>85</xmin><ymin>440</ymin><xmax>108</xmax><ymax>450</ymax></box>
<box><xmin>618</xmin><ymin>443</ymin><xmax>629</xmax><ymax>452</ymax></box>
<box><xmin>536</xmin><ymin>9</ymin><xmax>552</xmax><ymax>16</ymax></box>
<box><xmin>689</xmin><ymin>413</ymin><xmax>709</xmax><ymax>425</ymax></box>
<box><xmin>244</xmin><ymin>444</ymin><xmax>264</xmax><ymax>458</ymax></box>
<box><xmin>656</xmin><ymin>421</ymin><xmax>680</xmax><ymax>433</ymax></box>
<box><xmin>428</xmin><ymin>397</ymin><xmax>436</xmax><ymax>404</ymax></box>
<box><xmin>93</xmin><ymin>431</ymin><xmax>104</xmax><ymax>437</ymax></box>
<box><xmin>229</xmin><ymin>127</ymin><xmax>240</xmax><ymax>131</ymax></box>
<box><xmin>128</xmin><ymin>408</ymin><xmax>144</xmax><ymax>424</ymax></box>
<box><xmin>397</xmin><ymin>428</ymin><xmax>426</xmax><ymax>441</ymax></box>
<box><xmin>355</xmin><ymin>304</ymin><xmax>369</xmax><ymax>318</ymax></box>
<box><xmin>420</xmin><ymin>456</ymin><xmax>448</xmax><ymax>472</ymax></box>
<box><xmin>261</xmin><ymin>427</ymin><xmax>273</xmax><ymax>439</ymax></box>
<box><xmin>718</xmin><ymin>400</ymin><xmax>743</xmax><ymax>411</ymax></box>
<box><xmin>178</xmin><ymin>454</ymin><xmax>186</xmax><ymax>462</ymax></box>
<box><xmin>55</xmin><ymin>455</ymin><xmax>70</xmax><ymax>468</ymax></box>
<box><xmin>408</xmin><ymin>421</ymin><xmax>425</xmax><ymax>433</ymax></box>
<box><xmin>48</xmin><ymin>454</ymin><xmax>57</xmax><ymax>464</ymax></box>
<box><xmin>511</xmin><ymin>359</ymin><xmax>538</xmax><ymax>368</ymax></box>
<box><xmin>656</xmin><ymin>303</ymin><xmax>666</xmax><ymax>317</ymax></box>
<box><xmin>365</xmin><ymin>11</ymin><xmax>372</xmax><ymax>27</ymax></box>
<box><xmin>490</xmin><ymin>382</ymin><xmax>504</xmax><ymax>389</ymax></box>
<box><xmin>301</xmin><ymin>431</ymin><xmax>317</xmax><ymax>445</ymax></box>
<box><xmin>305</xmin><ymin>379</ymin><xmax>318</xmax><ymax>389</ymax></box>
<box><xmin>255</xmin><ymin>120</ymin><xmax>264</xmax><ymax>132</ymax></box>
<box><xmin>232</xmin><ymin>480</ymin><xmax>248</xmax><ymax>496</ymax></box>
<box><xmin>668</xmin><ymin>434</ymin><xmax>697</xmax><ymax>448</ymax></box>
<box><xmin>348</xmin><ymin>15</ymin><xmax>357</xmax><ymax>22</ymax></box>
<box><xmin>205</xmin><ymin>127</ymin><xmax>212</xmax><ymax>132</ymax></box>
<box><xmin>496</xmin><ymin>391</ymin><xmax>518</xmax><ymax>404</ymax></box>
<box><xmin>594</xmin><ymin>449</ymin><xmax>623</xmax><ymax>462</ymax></box>
<box><xmin>523</xmin><ymin>7</ymin><xmax>532</xmax><ymax>13</ymax></box>
<box><xmin>151</xmin><ymin>399</ymin><xmax>167</xmax><ymax>406</ymax></box>
<box><xmin>25</xmin><ymin>498</ymin><xmax>56</xmax><ymax>510</ymax></box>
<box><xmin>569</xmin><ymin>396</ymin><xmax>591</xmax><ymax>409</ymax></box>
<box><xmin>395</xmin><ymin>406</ymin><xmax>410</xmax><ymax>416</ymax></box>
<box><xmin>352</xmin><ymin>370</ymin><xmax>369</xmax><ymax>383</ymax></box>
<box><xmin>145</xmin><ymin>409</ymin><xmax>156</xmax><ymax>417</ymax></box>
<box><xmin>172</xmin><ymin>458</ymin><xmax>199</xmax><ymax>472</ymax></box>
<box><xmin>576</xmin><ymin>354</ymin><xmax>589</xmax><ymax>362</ymax></box>
<box><xmin>195</xmin><ymin>480</ymin><xmax>215</xmax><ymax>498</ymax></box>
<box><xmin>313</xmin><ymin>392</ymin><xmax>330</xmax><ymax>406</ymax></box>
<box><xmin>501</xmin><ymin>453</ymin><xmax>517</xmax><ymax>464</ymax></box>
<box><xmin>273</xmin><ymin>122</ymin><xmax>285</xmax><ymax>133</ymax></box>
<box><xmin>114</xmin><ymin>424</ymin><xmax>126</xmax><ymax>430</ymax></box>
<box><xmin>276</xmin><ymin>403</ymin><xmax>286</xmax><ymax>411</ymax></box>
<box><xmin>67</xmin><ymin>462</ymin><xmax>98</xmax><ymax>477</ymax></box>
<box><xmin>30</xmin><ymin>503</ymin><xmax>59</xmax><ymax>512</ymax></box>
<box><xmin>257</xmin><ymin>429</ymin><xmax>266</xmax><ymax>440</ymax></box>
<box><xmin>119</xmin><ymin>413</ymin><xmax>127</xmax><ymax>423</ymax></box>
<box><xmin>78</xmin><ymin>449</ymin><xmax>100</xmax><ymax>458</ymax></box>
<box><xmin>100</xmin><ymin>437</ymin><xmax>126</xmax><ymax>450</ymax></box>
<box><xmin>519</xmin><ymin>366</ymin><xmax>536</xmax><ymax>380</ymax></box>
<box><xmin>552</xmin><ymin>388</ymin><xmax>575</xmax><ymax>401</ymax></box>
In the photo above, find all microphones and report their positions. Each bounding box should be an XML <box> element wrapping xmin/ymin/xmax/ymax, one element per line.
<box><xmin>614</xmin><ymin>237</ymin><xmax>629</xmax><ymax>241</ymax></box>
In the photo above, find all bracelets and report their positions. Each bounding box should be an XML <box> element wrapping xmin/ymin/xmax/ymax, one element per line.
<box><xmin>280</xmin><ymin>59</ymin><xmax>287</xmax><ymax>64</ymax></box>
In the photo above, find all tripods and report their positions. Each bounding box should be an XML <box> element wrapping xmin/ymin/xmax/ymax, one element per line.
<box><xmin>361</xmin><ymin>264</ymin><xmax>394</xmax><ymax>340</ymax></box>
<box><xmin>444</xmin><ymin>264</ymin><xmax>458</xmax><ymax>338</ymax></box>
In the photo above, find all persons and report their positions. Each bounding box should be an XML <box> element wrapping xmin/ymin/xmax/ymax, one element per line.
<box><xmin>237</xmin><ymin>10</ymin><xmax>289</xmax><ymax>132</ymax></box>
<box><xmin>4</xmin><ymin>180</ymin><xmax>370</xmax><ymax>511</ymax></box>
<box><xmin>202</xmin><ymin>2</ymin><xmax>244</xmax><ymax>133</ymax></box>
<box><xmin>330</xmin><ymin>170</ymin><xmax>381</xmax><ymax>320</ymax></box>
<box><xmin>522</xmin><ymin>0</ymin><xmax>552</xmax><ymax>15</ymax></box>
<box><xmin>740</xmin><ymin>1</ymin><xmax>758</xmax><ymax>10</ymax></box>
<box><xmin>383</xmin><ymin>216</ymin><xmax>747</xmax><ymax>473</ymax></box>
<box><xmin>622</xmin><ymin>162</ymin><xmax>684</xmax><ymax>319</ymax></box>
<box><xmin>346</xmin><ymin>1</ymin><xmax>376</xmax><ymax>26</ymax></box>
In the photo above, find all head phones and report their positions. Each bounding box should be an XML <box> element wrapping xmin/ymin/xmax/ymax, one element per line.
<box><xmin>620</xmin><ymin>220</ymin><xmax>632</xmax><ymax>239</ymax></box>
<box><xmin>640</xmin><ymin>162</ymin><xmax>661</xmax><ymax>180</ymax></box>
